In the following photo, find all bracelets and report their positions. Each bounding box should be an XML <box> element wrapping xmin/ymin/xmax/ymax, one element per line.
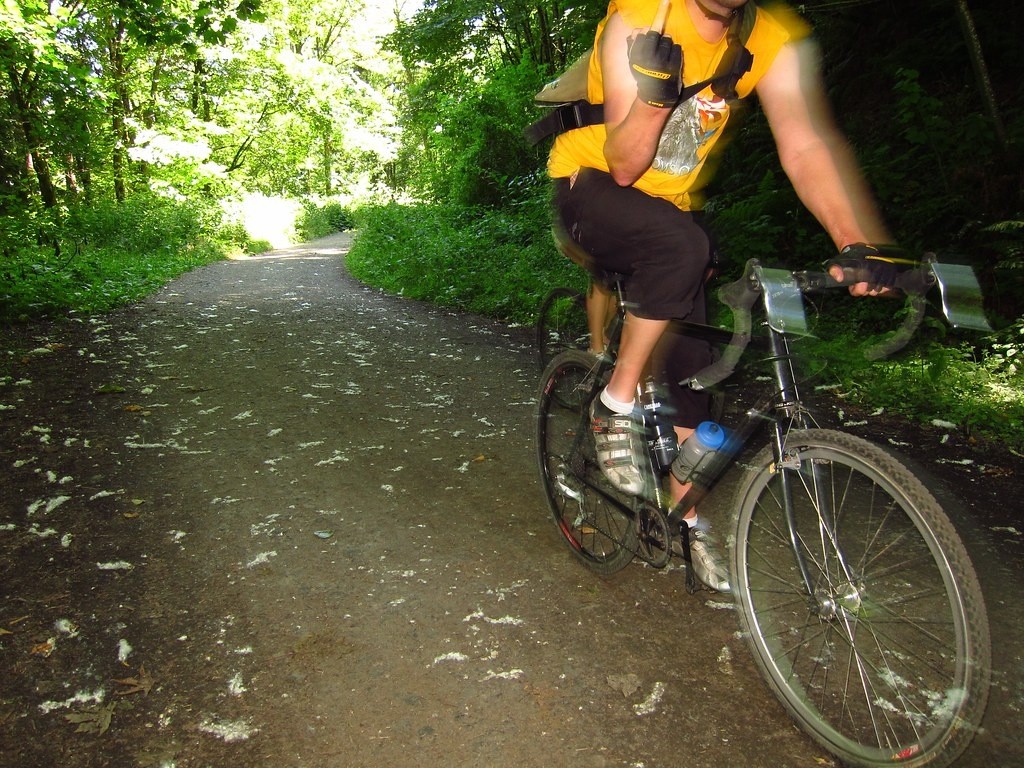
<box><xmin>636</xmin><ymin>91</ymin><xmax>679</xmax><ymax>108</ymax></box>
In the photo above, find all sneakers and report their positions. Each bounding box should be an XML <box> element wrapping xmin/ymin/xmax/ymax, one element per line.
<box><xmin>671</xmin><ymin>523</ymin><xmax>731</xmax><ymax>594</ymax></box>
<box><xmin>589</xmin><ymin>388</ymin><xmax>646</xmax><ymax>497</ymax></box>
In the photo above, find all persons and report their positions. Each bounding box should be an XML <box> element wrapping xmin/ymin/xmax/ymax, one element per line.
<box><xmin>542</xmin><ymin>0</ymin><xmax>912</xmax><ymax>595</ymax></box>
<box><xmin>546</xmin><ymin>197</ymin><xmax>622</xmax><ymax>365</ymax></box>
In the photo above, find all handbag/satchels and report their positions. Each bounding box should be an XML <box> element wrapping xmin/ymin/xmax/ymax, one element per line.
<box><xmin>534</xmin><ymin>44</ymin><xmax>594</xmax><ymax>107</ymax></box>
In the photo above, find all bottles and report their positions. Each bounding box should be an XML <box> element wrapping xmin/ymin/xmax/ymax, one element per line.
<box><xmin>672</xmin><ymin>421</ymin><xmax>726</xmax><ymax>484</ymax></box>
<box><xmin>641</xmin><ymin>376</ymin><xmax>679</xmax><ymax>468</ymax></box>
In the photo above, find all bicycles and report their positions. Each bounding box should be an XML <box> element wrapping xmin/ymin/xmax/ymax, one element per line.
<box><xmin>535</xmin><ymin>251</ymin><xmax>996</xmax><ymax>768</ymax></box>
<box><xmin>535</xmin><ymin>265</ymin><xmax>728</xmax><ymax>436</ymax></box>
